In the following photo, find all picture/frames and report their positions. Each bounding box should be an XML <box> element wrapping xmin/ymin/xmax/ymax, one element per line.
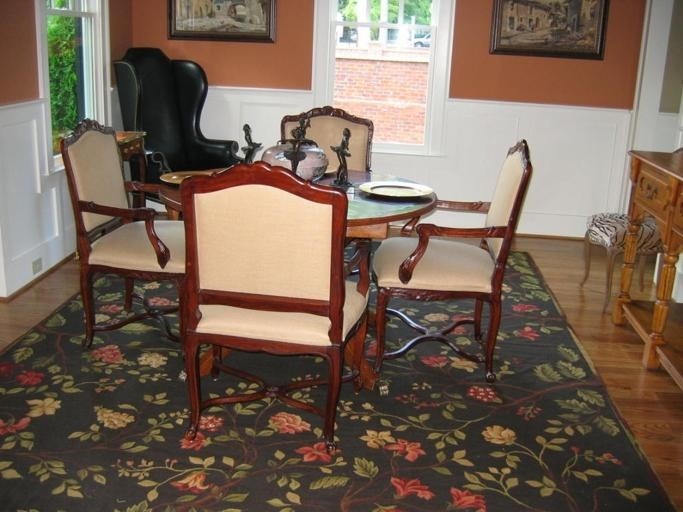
<box><xmin>489</xmin><ymin>2</ymin><xmax>608</xmax><ymax>62</ymax></box>
<box><xmin>167</xmin><ymin>0</ymin><xmax>277</xmax><ymax>45</ymax></box>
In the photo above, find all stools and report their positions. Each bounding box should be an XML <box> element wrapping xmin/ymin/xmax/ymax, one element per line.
<box><xmin>579</xmin><ymin>211</ymin><xmax>666</xmax><ymax>315</ymax></box>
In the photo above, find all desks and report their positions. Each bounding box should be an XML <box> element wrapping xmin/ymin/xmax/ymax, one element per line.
<box><xmin>104</xmin><ymin>128</ymin><xmax>151</xmax><ymax>214</ymax></box>
<box><xmin>152</xmin><ymin>165</ymin><xmax>441</xmax><ymax>398</ymax></box>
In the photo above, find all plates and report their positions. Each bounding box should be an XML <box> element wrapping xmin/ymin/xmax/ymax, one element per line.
<box><xmin>359</xmin><ymin>180</ymin><xmax>435</xmax><ymax>200</ymax></box>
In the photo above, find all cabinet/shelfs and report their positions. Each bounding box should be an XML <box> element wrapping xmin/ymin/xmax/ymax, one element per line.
<box><xmin>613</xmin><ymin>145</ymin><xmax>681</xmax><ymax>398</ymax></box>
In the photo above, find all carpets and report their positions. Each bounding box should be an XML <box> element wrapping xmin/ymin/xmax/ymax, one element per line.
<box><xmin>0</xmin><ymin>242</ymin><xmax>675</xmax><ymax>509</ymax></box>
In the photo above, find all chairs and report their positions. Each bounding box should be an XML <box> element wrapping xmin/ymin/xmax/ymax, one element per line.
<box><xmin>368</xmin><ymin>133</ymin><xmax>536</xmax><ymax>388</ymax></box>
<box><xmin>173</xmin><ymin>155</ymin><xmax>372</xmax><ymax>457</ymax></box>
<box><xmin>111</xmin><ymin>43</ymin><xmax>244</xmax><ymax>214</ymax></box>
<box><xmin>59</xmin><ymin>116</ymin><xmax>187</xmax><ymax>353</ymax></box>
<box><xmin>277</xmin><ymin>102</ymin><xmax>372</xmax><ymax>172</ymax></box>
